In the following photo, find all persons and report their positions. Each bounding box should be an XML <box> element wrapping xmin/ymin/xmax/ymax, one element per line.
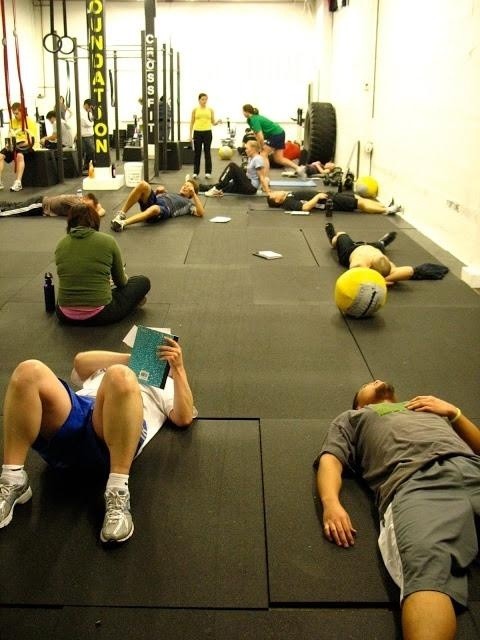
<box><xmin>189</xmin><ymin>93</ymin><xmax>224</xmax><ymax>178</ymax></box>
<box><xmin>131</xmin><ymin>95</ymin><xmax>172</xmax><ymax>145</ymax></box>
<box><xmin>0</xmin><ymin>95</ymin><xmax>95</xmax><ymax>191</ymax></box>
<box><xmin>54</xmin><ymin>203</ymin><xmax>151</xmax><ymax>328</ymax></box>
<box><xmin>200</xmin><ymin>104</ymin><xmax>402</xmax><ymax>217</ymax></box>
<box><xmin>111</xmin><ymin>179</ymin><xmax>204</xmax><ymax>233</ymax></box>
<box><xmin>325</xmin><ymin>221</ymin><xmax>415</xmax><ymax>287</ymax></box>
<box><xmin>0</xmin><ymin>335</ymin><xmax>195</xmax><ymax>545</ymax></box>
<box><xmin>1</xmin><ymin>192</ymin><xmax>106</xmax><ymax>218</ymax></box>
<box><xmin>311</xmin><ymin>380</ymin><xmax>479</xmax><ymax>640</ymax></box>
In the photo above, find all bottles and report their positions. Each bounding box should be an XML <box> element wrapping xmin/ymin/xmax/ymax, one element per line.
<box><xmin>338</xmin><ymin>178</ymin><xmax>342</xmax><ymax>193</ymax></box>
<box><xmin>325</xmin><ymin>199</ymin><xmax>333</xmax><ymax>217</ymax></box>
<box><xmin>88</xmin><ymin>160</ymin><xmax>95</xmax><ymax>178</ymax></box>
<box><xmin>131</xmin><ymin>132</ymin><xmax>143</xmax><ymax>147</ymax></box>
<box><xmin>42</xmin><ymin>272</ymin><xmax>56</xmax><ymax>312</ymax></box>
<box><xmin>111</xmin><ymin>163</ymin><xmax>116</xmax><ymax>177</ymax></box>
<box><xmin>76</xmin><ymin>187</ymin><xmax>82</xmax><ymax>197</ymax></box>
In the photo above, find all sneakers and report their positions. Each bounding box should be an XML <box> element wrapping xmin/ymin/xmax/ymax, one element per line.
<box><xmin>100</xmin><ymin>481</ymin><xmax>135</xmax><ymax>545</ymax></box>
<box><xmin>0</xmin><ymin>181</ymin><xmax>4</xmax><ymax>190</ymax></box>
<box><xmin>378</xmin><ymin>231</ymin><xmax>396</xmax><ymax>247</ymax></box>
<box><xmin>186</xmin><ymin>173</ymin><xmax>211</xmax><ymax>183</ymax></box>
<box><xmin>110</xmin><ymin>210</ymin><xmax>124</xmax><ymax>231</ymax></box>
<box><xmin>0</xmin><ymin>468</ymin><xmax>32</xmax><ymax>528</ymax></box>
<box><xmin>9</xmin><ymin>181</ymin><xmax>23</xmax><ymax>191</ymax></box>
<box><xmin>382</xmin><ymin>196</ymin><xmax>401</xmax><ymax>216</ymax></box>
<box><xmin>298</xmin><ymin>165</ymin><xmax>307</xmax><ymax>182</ymax></box>
<box><xmin>204</xmin><ymin>185</ymin><xmax>223</xmax><ymax>197</ymax></box>
<box><xmin>326</xmin><ymin>223</ymin><xmax>337</xmax><ymax>248</ymax></box>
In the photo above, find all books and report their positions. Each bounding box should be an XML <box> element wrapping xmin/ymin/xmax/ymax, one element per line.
<box><xmin>252</xmin><ymin>250</ymin><xmax>283</xmax><ymax>260</ymax></box>
<box><xmin>127</xmin><ymin>325</ymin><xmax>179</xmax><ymax>390</ymax></box>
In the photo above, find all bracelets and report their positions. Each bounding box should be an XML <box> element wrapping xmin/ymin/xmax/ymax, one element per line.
<box><xmin>450</xmin><ymin>408</ymin><xmax>461</xmax><ymax>424</ymax></box>
